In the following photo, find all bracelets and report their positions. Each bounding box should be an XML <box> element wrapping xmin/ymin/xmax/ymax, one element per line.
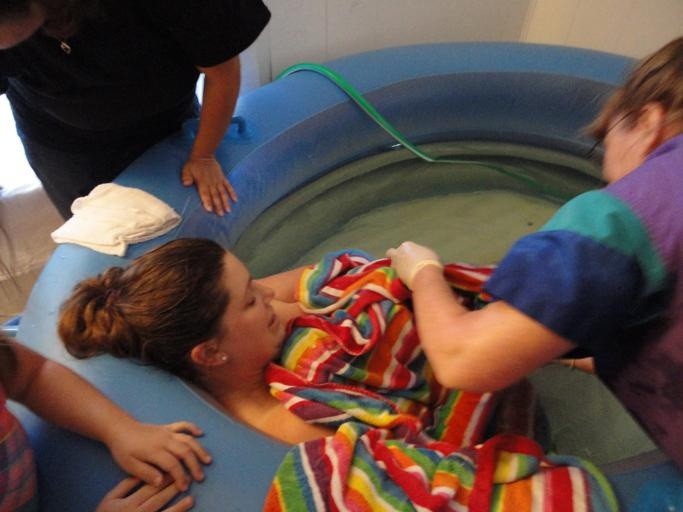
<box><xmin>404</xmin><ymin>258</ymin><xmax>444</xmax><ymax>291</ymax></box>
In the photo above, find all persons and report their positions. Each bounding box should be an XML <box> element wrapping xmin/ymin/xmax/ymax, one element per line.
<box><xmin>56</xmin><ymin>236</ymin><xmax>359</xmax><ymax>452</ymax></box>
<box><xmin>0</xmin><ymin>333</ymin><xmax>211</xmax><ymax>511</ymax></box>
<box><xmin>0</xmin><ymin>0</ymin><xmax>270</xmax><ymax>217</ymax></box>
<box><xmin>383</xmin><ymin>33</ymin><xmax>681</xmax><ymax>477</ymax></box>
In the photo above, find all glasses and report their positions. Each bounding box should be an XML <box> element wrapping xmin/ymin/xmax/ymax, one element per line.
<box><xmin>589</xmin><ymin>111</ymin><xmax>632</xmax><ymax>169</ymax></box>
